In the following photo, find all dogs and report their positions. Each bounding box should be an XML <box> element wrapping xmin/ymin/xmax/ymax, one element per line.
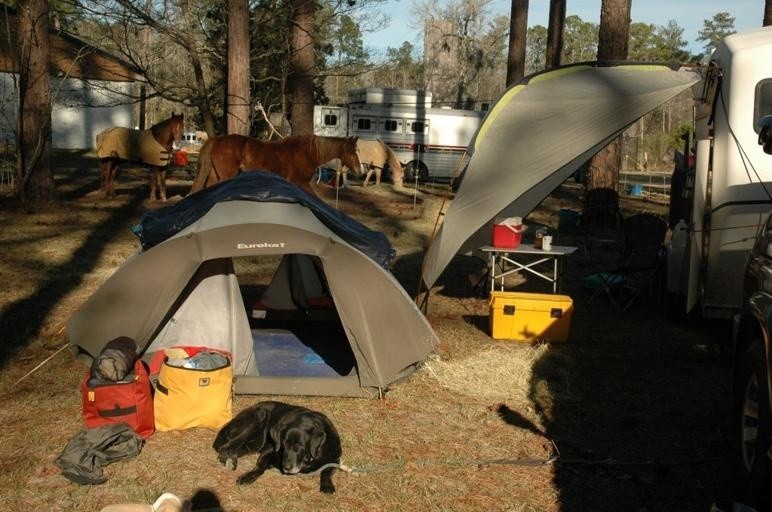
<box><xmin>211</xmin><ymin>400</ymin><xmax>344</xmax><ymax>497</ymax></box>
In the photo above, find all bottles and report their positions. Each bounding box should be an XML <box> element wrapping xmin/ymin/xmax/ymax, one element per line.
<box><xmin>535</xmin><ymin>225</ymin><xmax>548</xmax><ymax>249</ymax></box>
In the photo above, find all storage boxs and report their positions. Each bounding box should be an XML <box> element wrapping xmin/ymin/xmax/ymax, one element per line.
<box><xmin>486</xmin><ymin>291</ymin><xmax>575</xmax><ymax>343</ymax></box>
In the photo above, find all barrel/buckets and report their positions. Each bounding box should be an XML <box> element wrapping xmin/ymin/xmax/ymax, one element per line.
<box><xmin>175</xmin><ymin>151</ymin><xmax>188</xmax><ymax>165</ymax></box>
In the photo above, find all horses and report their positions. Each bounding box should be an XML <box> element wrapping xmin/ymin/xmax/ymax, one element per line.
<box><xmin>190</xmin><ymin>134</ymin><xmax>362</xmax><ymax>198</ymax></box>
<box><xmin>95</xmin><ymin>110</ymin><xmax>186</xmax><ymax>203</ymax></box>
<box><xmin>341</xmin><ymin>138</ymin><xmax>407</xmax><ymax>189</ymax></box>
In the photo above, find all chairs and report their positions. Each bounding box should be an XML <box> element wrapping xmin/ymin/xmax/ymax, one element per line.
<box><xmin>558</xmin><ymin>188</ymin><xmax>667</xmax><ymax>314</ymax></box>
<box><xmin>443</xmin><ymin>254</ymin><xmax>489</xmax><ymax>298</ymax></box>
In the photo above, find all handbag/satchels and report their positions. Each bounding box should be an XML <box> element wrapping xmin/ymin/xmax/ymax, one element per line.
<box><xmin>79</xmin><ymin>359</ymin><xmax>156</xmax><ymax>439</ymax></box>
<box><xmin>153</xmin><ymin>347</ymin><xmax>233</xmax><ymax>432</ymax></box>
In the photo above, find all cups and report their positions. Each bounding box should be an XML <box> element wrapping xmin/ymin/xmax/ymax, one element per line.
<box><xmin>542</xmin><ymin>236</ymin><xmax>553</xmax><ymax>251</ymax></box>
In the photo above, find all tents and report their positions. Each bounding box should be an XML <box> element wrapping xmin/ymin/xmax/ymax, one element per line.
<box><xmin>131</xmin><ymin>168</ymin><xmax>396</xmax><ymax>270</ymax></box>
<box><xmin>59</xmin><ymin>201</ymin><xmax>441</xmax><ymax>404</ymax></box>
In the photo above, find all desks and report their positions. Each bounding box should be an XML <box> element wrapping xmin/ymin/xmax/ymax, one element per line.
<box><xmin>477</xmin><ymin>243</ymin><xmax>579</xmax><ymax>295</ymax></box>
